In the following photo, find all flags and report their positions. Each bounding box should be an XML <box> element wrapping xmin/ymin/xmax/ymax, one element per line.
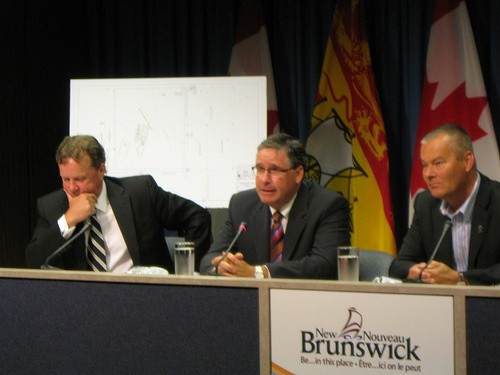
<box><xmin>213</xmin><ymin>0</ymin><xmax>500</xmax><ymax>260</ymax></box>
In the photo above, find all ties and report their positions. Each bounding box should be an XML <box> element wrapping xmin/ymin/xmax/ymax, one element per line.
<box><xmin>86</xmin><ymin>210</ymin><xmax>107</xmax><ymax>273</ymax></box>
<box><xmin>268</xmin><ymin>210</ymin><xmax>285</xmax><ymax>264</ymax></box>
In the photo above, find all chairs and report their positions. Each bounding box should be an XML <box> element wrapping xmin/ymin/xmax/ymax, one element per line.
<box><xmin>354</xmin><ymin>250</ymin><xmax>396</xmax><ymax>280</ymax></box>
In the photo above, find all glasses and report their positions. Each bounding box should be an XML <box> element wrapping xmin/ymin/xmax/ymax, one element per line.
<box><xmin>251</xmin><ymin>164</ymin><xmax>295</xmax><ymax>177</ymax></box>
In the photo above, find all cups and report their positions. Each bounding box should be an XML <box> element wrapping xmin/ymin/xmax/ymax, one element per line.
<box><xmin>174</xmin><ymin>241</ymin><xmax>195</xmax><ymax>277</ymax></box>
<box><xmin>337</xmin><ymin>246</ymin><xmax>360</xmax><ymax>282</ymax></box>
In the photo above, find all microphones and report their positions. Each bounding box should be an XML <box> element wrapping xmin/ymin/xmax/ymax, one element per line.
<box><xmin>207</xmin><ymin>221</ymin><xmax>248</xmax><ymax>276</ymax></box>
<box><xmin>419</xmin><ymin>221</ymin><xmax>453</xmax><ymax>283</ymax></box>
<box><xmin>41</xmin><ymin>223</ymin><xmax>93</xmax><ymax>270</ymax></box>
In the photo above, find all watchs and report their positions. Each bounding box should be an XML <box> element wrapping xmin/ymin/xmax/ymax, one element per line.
<box><xmin>255</xmin><ymin>266</ymin><xmax>265</xmax><ymax>279</ymax></box>
<box><xmin>457</xmin><ymin>273</ymin><xmax>466</xmax><ymax>286</ymax></box>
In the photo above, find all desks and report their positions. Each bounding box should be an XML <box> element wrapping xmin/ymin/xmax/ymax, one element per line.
<box><xmin>0</xmin><ymin>268</ymin><xmax>500</xmax><ymax>375</ymax></box>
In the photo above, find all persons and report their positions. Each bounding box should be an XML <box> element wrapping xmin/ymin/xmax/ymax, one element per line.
<box><xmin>23</xmin><ymin>135</ymin><xmax>213</xmax><ymax>274</ymax></box>
<box><xmin>388</xmin><ymin>123</ymin><xmax>500</xmax><ymax>286</ymax></box>
<box><xmin>201</xmin><ymin>133</ymin><xmax>350</xmax><ymax>279</ymax></box>
